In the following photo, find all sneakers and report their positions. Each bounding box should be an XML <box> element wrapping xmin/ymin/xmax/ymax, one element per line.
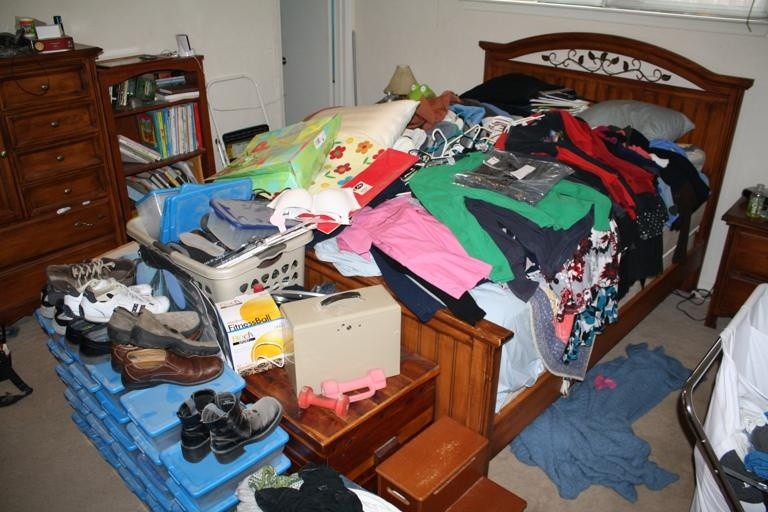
<box><xmin>38</xmin><ymin>258</ymin><xmax>222</xmax><ymax>389</ymax></box>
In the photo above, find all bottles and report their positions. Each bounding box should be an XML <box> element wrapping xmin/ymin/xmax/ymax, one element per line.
<box><xmin>746</xmin><ymin>184</ymin><xmax>766</xmax><ymax>218</ymax></box>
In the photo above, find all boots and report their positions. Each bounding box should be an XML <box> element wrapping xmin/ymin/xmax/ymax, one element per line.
<box><xmin>200</xmin><ymin>393</ymin><xmax>283</xmax><ymax>464</ymax></box>
<box><xmin>177</xmin><ymin>389</ymin><xmax>217</xmax><ymax>462</ymax></box>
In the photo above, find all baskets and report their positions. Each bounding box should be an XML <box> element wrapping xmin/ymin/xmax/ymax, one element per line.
<box><xmin>126</xmin><ymin>214</ymin><xmax>314</xmax><ymax>304</ymax></box>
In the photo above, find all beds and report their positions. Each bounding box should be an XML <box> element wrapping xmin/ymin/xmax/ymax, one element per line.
<box><xmin>304</xmin><ymin>32</ymin><xmax>755</xmax><ymax>460</ymax></box>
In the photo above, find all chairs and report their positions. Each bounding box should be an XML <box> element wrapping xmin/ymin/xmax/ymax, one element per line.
<box><xmin>205</xmin><ymin>74</ymin><xmax>271</xmax><ymax>169</ymax></box>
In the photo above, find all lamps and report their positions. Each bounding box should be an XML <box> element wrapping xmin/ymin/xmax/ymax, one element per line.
<box><xmin>382</xmin><ymin>61</ymin><xmax>419</xmax><ymax>99</ymax></box>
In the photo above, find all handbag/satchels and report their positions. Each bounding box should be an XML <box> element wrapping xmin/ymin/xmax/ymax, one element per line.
<box><xmin>204</xmin><ymin>113</ymin><xmax>341</xmax><ymax>191</ymax></box>
<box><xmin>257</xmin><ymin>134</ymin><xmax>421</xmax><ymax>235</ymax></box>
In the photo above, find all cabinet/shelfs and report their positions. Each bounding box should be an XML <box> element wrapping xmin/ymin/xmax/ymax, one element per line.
<box><xmin>240</xmin><ymin>346</ymin><xmax>442</xmax><ymax>494</ymax></box>
<box><xmin>0</xmin><ymin>40</ymin><xmax>127</xmax><ymax>329</ymax></box>
<box><xmin>97</xmin><ymin>51</ymin><xmax>214</xmax><ymax>240</ymax></box>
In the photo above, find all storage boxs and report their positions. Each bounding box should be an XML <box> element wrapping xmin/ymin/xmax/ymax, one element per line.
<box><xmin>207</xmin><ymin>196</ymin><xmax>300</xmax><ymax>250</ymax></box>
<box><xmin>135</xmin><ymin>186</ymin><xmax>179</xmax><ymax>240</ymax></box>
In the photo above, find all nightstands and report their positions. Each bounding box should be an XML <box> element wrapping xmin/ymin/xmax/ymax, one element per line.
<box><xmin>704</xmin><ymin>188</ymin><xmax>768</xmax><ymax>330</ymax></box>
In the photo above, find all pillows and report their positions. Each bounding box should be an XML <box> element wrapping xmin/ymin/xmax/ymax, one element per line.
<box><xmin>310</xmin><ymin>100</ymin><xmax>419</xmax><ymax>152</ymax></box>
<box><xmin>578</xmin><ymin>100</ymin><xmax>695</xmax><ymax>144</ymax></box>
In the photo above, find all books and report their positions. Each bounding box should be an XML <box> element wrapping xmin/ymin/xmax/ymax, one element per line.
<box><xmin>108</xmin><ymin>69</ymin><xmax>208</xmax><ymax>211</ymax></box>
